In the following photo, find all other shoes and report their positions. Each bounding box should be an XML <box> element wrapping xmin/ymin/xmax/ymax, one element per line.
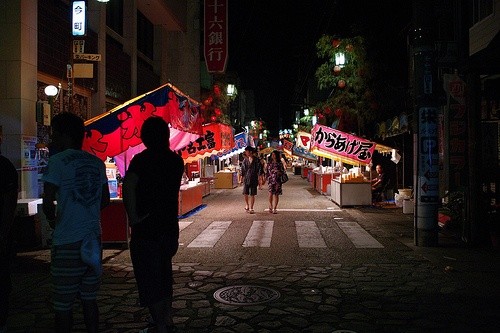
<box><xmin>249</xmin><ymin>209</ymin><xmax>254</xmax><ymax>214</ymax></box>
<box><xmin>269</xmin><ymin>206</ymin><xmax>272</xmax><ymax>213</ymax></box>
<box><xmin>273</xmin><ymin>209</ymin><xmax>277</xmax><ymax>214</ymax></box>
<box><xmin>245</xmin><ymin>204</ymin><xmax>249</xmax><ymax>212</ymax></box>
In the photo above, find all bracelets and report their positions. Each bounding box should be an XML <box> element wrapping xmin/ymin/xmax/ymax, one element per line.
<box><xmin>46</xmin><ymin>215</ymin><xmax>57</xmax><ymax>222</ymax></box>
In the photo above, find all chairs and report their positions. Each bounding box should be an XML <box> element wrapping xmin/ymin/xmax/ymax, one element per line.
<box><xmin>372</xmin><ymin>178</ymin><xmax>392</xmax><ymax>207</ymax></box>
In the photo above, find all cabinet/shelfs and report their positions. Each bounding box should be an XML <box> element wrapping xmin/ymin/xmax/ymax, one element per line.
<box><xmin>331</xmin><ymin>177</ymin><xmax>372</xmax><ymax>207</ymax></box>
<box><xmin>100</xmin><ymin>197</ymin><xmax>131</xmax><ymax>250</ymax></box>
<box><xmin>213</xmin><ymin>171</ymin><xmax>240</xmax><ymax>189</ymax></box>
<box><xmin>308</xmin><ymin>169</ymin><xmax>340</xmax><ymax>195</ymax></box>
<box><xmin>292</xmin><ymin>166</ymin><xmax>301</xmax><ymax>175</ymax></box>
<box><xmin>177</xmin><ymin>179</ymin><xmax>210</xmax><ymax>216</ymax></box>
<box><xmin>301</xmin><ymin>166</ymin><xmax>313</xmax><ymax>179</ymax></box>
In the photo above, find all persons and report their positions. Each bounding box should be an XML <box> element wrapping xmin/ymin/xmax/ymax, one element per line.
<box><xmin>372</xmin><ymin>163</ymin><xmax>386</xmax><ymax>200</ymax></box>
<box><xmin>262</xmin><ymin>149</ymin><xmax>286</xmax><ymax>214</ymax></box>
<box><xmin>122</xmin><ymin>116</ymin><xmax>185</xmax><ymax>333</ymax></box>
<box><xmin>252</xmin><ymin>150</ymin><xmax>310</xmax><ymax>190</ymax></box>
<box><xmin>239</xmin><ymin>146</ymin><xmax>266</xmax><ymax>214</ymax></box>
<box><xmin>37</xmin><ymin>109</ymin><xmax>110</xmax><ymax>333</ymax></box>
<box><xmin>0</xmin><ymin>123</ymin><xmax>22</xmax><ymax>332</ymax></box>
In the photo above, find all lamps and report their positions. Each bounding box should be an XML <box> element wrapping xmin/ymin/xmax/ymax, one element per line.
<box><xmin>45</xmin><ymin>84</ymin><xmax>58</xmax><ymax>104</ymax></box>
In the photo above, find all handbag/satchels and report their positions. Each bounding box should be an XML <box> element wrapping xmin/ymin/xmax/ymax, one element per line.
<box><xmin>279</xmin><ymin>173</ymin><xmax>288</xmax><ymax>184</ymax></box>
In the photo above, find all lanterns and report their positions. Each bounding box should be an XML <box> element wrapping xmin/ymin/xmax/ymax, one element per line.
<box><xmin>198</xmin><ymin>37</ymin><xmax>381</xmax><ymax>130</ymax></box>
